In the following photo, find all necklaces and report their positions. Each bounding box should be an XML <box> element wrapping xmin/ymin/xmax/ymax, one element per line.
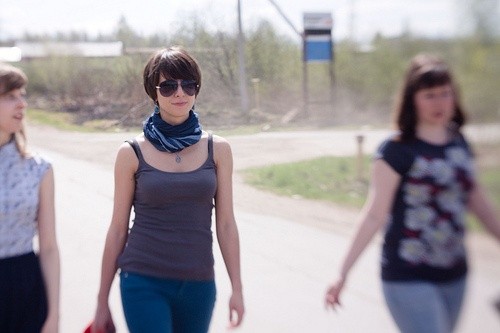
<box><xmin>174</xmin><ymin>151</ymin><xmax>186</xmax><ymax>164</ymax></box>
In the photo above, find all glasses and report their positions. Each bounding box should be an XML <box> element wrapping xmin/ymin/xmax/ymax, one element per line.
<box><xmin>155</xmin><ymin>79</ymin><xmax>200</xmax><ymax>97</ymax></box>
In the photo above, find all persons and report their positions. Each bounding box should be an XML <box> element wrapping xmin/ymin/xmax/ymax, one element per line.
<box><xmin>318</xmin><ymin>51</ymin><xmax>500</xmax><ymax>333</ymax></box>
<box><xmin>0</xmin><ymin>62</ymin><xmax>64</xmax><ymax>333</ymax></box>
<box><xmin>85</xmin><ymin>46</ymin><xmax>246</xmax><ymax>333</ymax></box>
